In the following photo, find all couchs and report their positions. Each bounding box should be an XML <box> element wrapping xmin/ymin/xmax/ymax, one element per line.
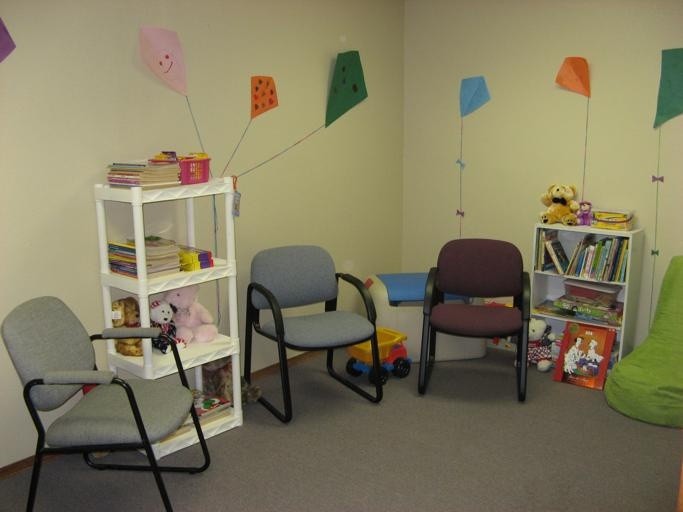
<box><xmin>604</xmin><ymin>256</ymin><xmax>682</xmax><ymax>427</ymax></box>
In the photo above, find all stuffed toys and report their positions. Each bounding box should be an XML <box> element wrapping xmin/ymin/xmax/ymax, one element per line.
<box><xmin>514</xmin><ymin>318</ymin><xmax>556</xmax><ymax>372</ymax></box>
<box><xmin>149</xmin><ymin>301</ymin><xmax>185</xmax><ymax>355</ymax></box>
<box><xmin>112</xmin><ymin>296</ymin><xmax>142</xmax><ymax>357</ymax></box>
<box><xmin>540</xmin><ymin>184</ymin><xmax>579</xmax><ymax>225</ymax></box>
<box><xmin>166</xmin><ymin>283</ymin><xmax>218</xmax><ymax>348</ymax></box>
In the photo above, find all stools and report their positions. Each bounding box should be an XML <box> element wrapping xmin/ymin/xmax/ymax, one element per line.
<box><xmin>366</xmin><ymin>271</ymin><xmax>488</xmax><ymax>361</ymax></box>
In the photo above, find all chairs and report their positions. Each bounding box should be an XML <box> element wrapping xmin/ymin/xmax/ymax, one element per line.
<box><xmin>242</xmin><ymin>244</ymin><xmax>382</xmax><ymax>422</ymax></box>
<box><xmin>418</xmin><ymin>238</ymin><xmax>532</xmax><ymax>401</ymax></box>
<box><xmin>3</xmin><ymin>298</ymin><xmax>211</xmax><ymax>512</ymax></box>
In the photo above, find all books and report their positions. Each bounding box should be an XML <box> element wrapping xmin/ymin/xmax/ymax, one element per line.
<box><xmin>565</xmin><ymin>235</ymin><xmax>628</xmax><ymax>283</ymax></box>
<box><xmin>193</xmin><ymin>387</ymin><xmax>230</xmax><ymax>419</ymax></box>
<box><xmin>536</xmin><ymin>229</ymin><xmax>570</xmax><ymax>273</ymax></box>
<box><xmin>107</xmin><ymin>162</ymin><xmax>181</xmax><ymax>189</ymax></box>
<box><xmin>109</xmin><ymin>234</ymin><xmax>182</xmax><ymax>279</ymax></box>
<box><xmin>178</xmin><ymin>244</ymin><xmax>214</xmax><ymax>271</ymax></box>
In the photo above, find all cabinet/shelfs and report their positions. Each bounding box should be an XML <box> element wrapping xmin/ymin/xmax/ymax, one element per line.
<box><xmin>92</xmin><ymin>175</ymin><xmax>242</xmax><ymax>458</ymax></box>
<box><xmin>528</xmin><ymin>223</ymin><xmax>641</xmax><ymax>378</ymax></box>
